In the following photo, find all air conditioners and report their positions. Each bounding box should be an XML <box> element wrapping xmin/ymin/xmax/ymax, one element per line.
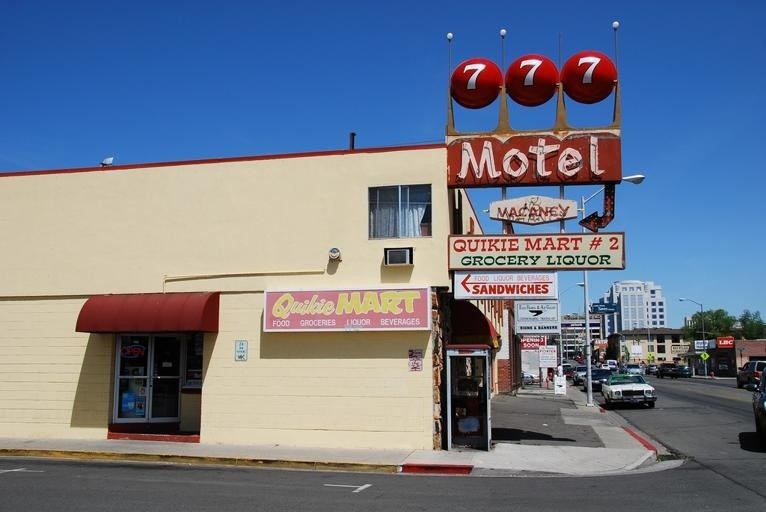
<box><xmin>387</xmin><ymin>249</ymin><xmax>410</xmax><ymax>265</ymax></box>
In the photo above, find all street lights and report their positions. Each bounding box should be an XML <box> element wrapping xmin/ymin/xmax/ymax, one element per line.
<box><xmin>558</xmin><ymin>282</ymin><xmax>586</xmax><ymax>364</ymax></box>
<box><xmin>579</xmin><ymin>172</ymin><xmax>645</xmax><ymax>408</ymax></box>
<box><xmin>679</xmin><ymin>297</ymin><xmax>707</xmax><ymax>379</ymax></box>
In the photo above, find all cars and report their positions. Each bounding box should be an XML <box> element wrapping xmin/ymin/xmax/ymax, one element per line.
<box><xmin>522</xmin><ymin>355</ymin><xmax>693</xmax><ymax>408</ymax></box>
<box><xmin>753</xmin><ymin>367</ymin><xmax>766</xmax><ymax>438</ymax></box>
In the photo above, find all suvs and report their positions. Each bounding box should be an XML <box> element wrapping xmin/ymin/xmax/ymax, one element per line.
<box><xmin>737</xmin><ymin>360</ymin><xmax>766</xmax><ymax>390</ymax></box>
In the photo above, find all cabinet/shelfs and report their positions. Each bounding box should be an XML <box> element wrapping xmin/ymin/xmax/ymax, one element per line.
<box><xmin>451</xmin><ymin>392</ymin><xmax>482</xmax><ymax>436</ymax></box>
